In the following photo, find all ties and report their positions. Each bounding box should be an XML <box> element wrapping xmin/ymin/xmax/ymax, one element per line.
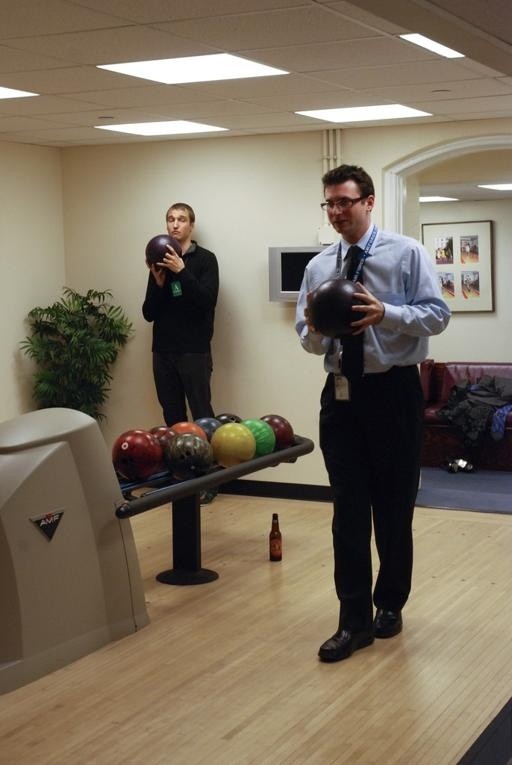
<box><xmin>341</xmin><ymin>249</ymin><xmax>365</xmax><ymax>384</ymax></box>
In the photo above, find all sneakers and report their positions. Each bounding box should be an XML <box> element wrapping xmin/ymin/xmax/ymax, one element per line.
<box><xmin>199</xmin><ymin>489</ymin><xmax>218</xmax><ymax>503</ymax></box>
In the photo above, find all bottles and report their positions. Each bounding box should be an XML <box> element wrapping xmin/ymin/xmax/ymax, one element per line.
<box><xmin>268</xmin><ymin>513</ymin><xmax>283</xmax><ymax>562</ymax></box>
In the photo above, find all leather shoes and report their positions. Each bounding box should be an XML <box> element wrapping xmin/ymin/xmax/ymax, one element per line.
<box><xmin>375</xmin><ymin>605</ymin><xmax>403</xmax><ymax>637</ymax></box>
<box><xmin>319</xmin><ymin>617</ymin><xmax>375</xmax><ymax>661</ymax></box>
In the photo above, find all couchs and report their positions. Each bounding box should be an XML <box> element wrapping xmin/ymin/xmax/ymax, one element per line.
<box><xmin>417</xmin><ymin>358</ymin><xmax>511</xmax><ymax>469</ymax></box>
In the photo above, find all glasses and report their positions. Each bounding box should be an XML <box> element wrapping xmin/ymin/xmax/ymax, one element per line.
<box><xmin>320</xmin><ymin>197</ymin><xmax>367</xmax><ymax>208</ymax></box>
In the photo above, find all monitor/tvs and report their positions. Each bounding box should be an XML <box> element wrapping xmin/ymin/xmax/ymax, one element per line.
<box><xmin>269</xmin><ymin>247</ymin><xmax>329</xmax><ymax>302</ymax></box>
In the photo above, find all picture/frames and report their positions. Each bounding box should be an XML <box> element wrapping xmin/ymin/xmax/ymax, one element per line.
<box><xmin>419</xmin><ymin>217</ymin><xmax>496</xmax><ymax>316</ymax></box>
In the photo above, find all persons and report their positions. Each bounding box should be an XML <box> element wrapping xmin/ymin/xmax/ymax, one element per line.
<box><xmin>143</xmin><ymin>203</ymin><xmax>220</xmax><ymax>505</ymax></box>
<box><xmin>295</xmin><ymin>164</ymin><xmax>452</xmax><ymax>663</ymax></box>
